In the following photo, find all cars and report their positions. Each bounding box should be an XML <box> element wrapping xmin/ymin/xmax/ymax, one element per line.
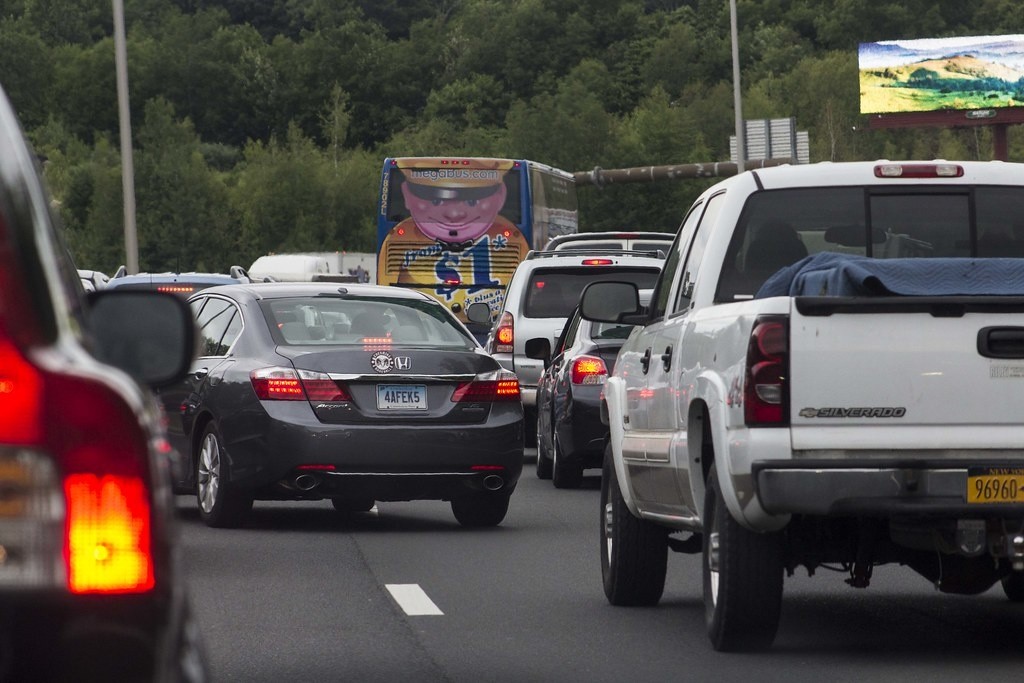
<box><xmin>525</xmin><ymin>289</ymin><xmax>655</xmax><ymax>487</ymax></box>
<box><xmin>0</xmin><ymin>82</ymin><xmax>211</xmax><ymax>683</ymax></box>
<box><xmin>159</xmin><ymin>282</ymin><xmax>525</xmax><ymax>529</ymax></box>
<box><xmin>76</xmin><ymin>269</ymin><xmax>111</xmax><ymax>293</ymax></box>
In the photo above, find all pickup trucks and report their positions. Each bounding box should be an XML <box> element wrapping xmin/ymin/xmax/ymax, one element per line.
<box><xmin>579</xmin><ymin>158</ymin><xmax>1024</xmax><ymax>653</ymax></box>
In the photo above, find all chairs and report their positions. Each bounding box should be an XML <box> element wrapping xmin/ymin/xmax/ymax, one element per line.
<box><xmin>280</xmin><ymin>322</ymin><xmax>312</xmax><ymax>340</ymax></box>
<box><xmin>308</xmin><ymin>325</ymin><xmax>327</xmax><ymax>340</ymax></box>
<box><xmin>720</xmin><ymin>237</ymin><xmax>808</xmax><ymax>297</ymax></box>
<box><xmin>391</xmin><ymin>325</ymin><xmax>422</xmax><ymax>344</ymax></box>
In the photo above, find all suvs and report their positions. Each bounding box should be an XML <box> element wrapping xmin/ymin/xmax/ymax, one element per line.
<box><xmin>467</xmin><ymin>250</ymin><xmax>667</xmax><ymax>447</ymax></box>
<box><xmin>105</xmin><ymin>265</ymin><xmax>251</xmax><ymax>300</ymax></box>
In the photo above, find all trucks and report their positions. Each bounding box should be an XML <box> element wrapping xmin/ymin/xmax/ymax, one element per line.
<box><xmin>542</xmin><ymin>232</ymin><xmax>675</xmax><ymax>260</ymax></box>
<box><xmin>377</xmin><ymin>157</ymin><xmax>579</xmax><ymax>349</ymax></box>
<box><xmin>247</xmin><ymin>252</ymin><xmax>377</xmax><ymax>285</ymax></box>
